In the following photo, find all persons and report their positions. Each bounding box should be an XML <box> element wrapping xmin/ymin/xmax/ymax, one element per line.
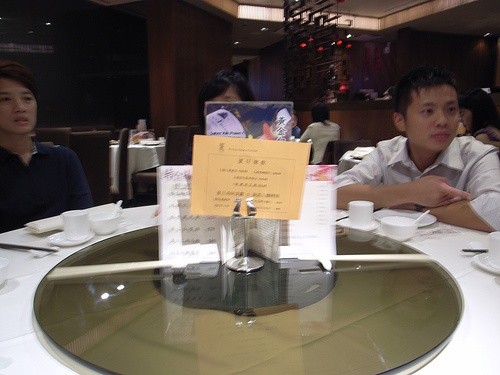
<box><xmin>0</xmin><ymin>60</ymin><xmax>93</xmax><ymax>234</ymax></box>
<box><xmin>334</xmin><ymin>62</ymin><xmax>500</xmax><ymax>233</ymax></box>
<box><xmin>198</xmin><ymin>70</ymin><xmax>254</xmax><ymax>117</ymax></box>
<box><xmin>291</xmin><ymin>110</ymin><xmax>301</xmax><ymax>138</ymax></box>
<box><xmin>460</xmin><ymin>89</ymin><xmax>500</xmax><ymax>144</ymax></box>
<box><xmin>300</xmin><ymin>103</ymin><xmax>340</xmax><ymax>164</ymax></box>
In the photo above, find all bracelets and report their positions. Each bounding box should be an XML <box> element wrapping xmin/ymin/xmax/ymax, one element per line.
<box><xmin>415</xmin><ymin>204</ymin><xmax>424</xmax><ymax>211</ymax></box>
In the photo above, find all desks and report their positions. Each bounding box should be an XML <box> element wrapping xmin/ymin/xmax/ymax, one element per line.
<box><xmin>110</xmin><ymin>138</ymin><xmax>165</xmax><ymax>194</ymax></box>
<box><xmin>0</xmin><ymin>200</ymin><xmax>500</xmax><ymax>375</ymax></box>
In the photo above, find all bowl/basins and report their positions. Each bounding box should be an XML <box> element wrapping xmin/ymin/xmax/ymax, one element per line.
<box><xmin>91</xmin><ymin>212</ymin><xmax>122</xmax><ymax>236</ymax></box>
<box><xmin>382</xmin><ymin>216</ymin><xmax>417</xmax><ymax>242</ymax></box>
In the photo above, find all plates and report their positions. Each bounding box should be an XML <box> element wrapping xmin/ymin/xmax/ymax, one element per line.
<box><xmin>338</xmin><ymin>216</ymin><xmax>381</xmax><ymax>232</ymax></box>
<box><xmin>141</xmin><ymin>142</ymin><xmax>159</xmax><ymax>145</ymax></box>
<box><xmin>472</xmin><ymin>253</ymin><xmax>500</xmax><ymax>275</ymax></box>
<box><xmin>373</xmin><ymin>207</ymin><xmax>436</xmax><ymax>228</ymax></box>
<box><xmin>47</xmin><ymin>231</ymin><xmax>94</xmax><ymax>246</ymax></box>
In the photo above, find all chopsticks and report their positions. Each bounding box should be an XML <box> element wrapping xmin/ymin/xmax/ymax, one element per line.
<box><xmin>462</xmin><ymin>249</ymin><xmax>488</xmax><ymax>252</ymax></box>
<box><xmin>0</xmin><ymin>243</ymin><xmax>58</xmax><ymax>252</ymax></box>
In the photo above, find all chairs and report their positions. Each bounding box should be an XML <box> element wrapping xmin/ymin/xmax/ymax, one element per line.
<box><xmin>315</xmin><ymin>139</ymin><xmax>368</xmax><ymax>166</ymax></box>
<box><xmin>35</xmin><ymin>123</ymin><xmax>202</xmax><ymax>206</ymax></box>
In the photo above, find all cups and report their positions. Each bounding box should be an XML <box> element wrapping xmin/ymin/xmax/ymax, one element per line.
<box><xmin>62</xmin><ymin>210</ymin><xmax>91</xmax><ymax>240</ymax></box>
<box><xmin>348</xmin><ymin>200</ymin><xmax>374</xmax><ymax>225</ymax></box>
<box><xmin>487</xmin><ymin>231</ymin><xmax>500</xmax><ymax>268</ymax></box>
